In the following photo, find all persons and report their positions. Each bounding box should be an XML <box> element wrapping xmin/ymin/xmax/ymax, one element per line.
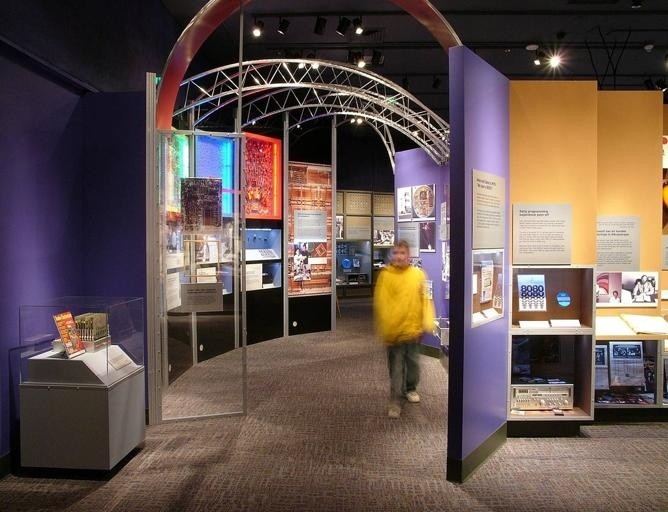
<box><xmin>299</xmin><ymin>244</ymin><xmax>308</xmax><ymax>264</ymax></box>
<box><xmin>294</xmin><ymin>248</ymin><xmax>307</xmax><ymax>265</ymax></box>
<box><xmin>609</xmin><ymin>291</ymin><xmax>621</xmax><ymax>302</ymax></box>
<box><xmin>632</xmin><ymin>274</ymin><xmax>655</xmax><ymax>302</ymax></box>
<box><xmin>372</xmin><ymin>239</ymin><xmax>437</xmax><ymax>418</ymax></box>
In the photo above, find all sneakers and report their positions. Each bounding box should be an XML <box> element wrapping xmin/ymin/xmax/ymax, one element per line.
<box><xmin>388</xmin><ymin>404</ymin><xmax>400</xmax><ymax>419</ymax></box>
<box><xmin>401</xmin><ymin>390</ymin><xmax>420</xmax><ymax>404</ymax></box>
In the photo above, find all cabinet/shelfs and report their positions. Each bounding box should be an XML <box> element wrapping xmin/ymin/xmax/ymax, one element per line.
<box><xmin>336</xmin><ymin>241</ymin><xmax>370</xmax><ymax>298</ymax></box>
<box><xmin>165</xmin><ymin>217</ymin><xmax>283</xmax><ymax>314</ymax></box>
<box><xmin>596</xmin><ymin>334</ymin><xmax>668</xmax><ymax>410</ymax></box>
<box><xmin>507</xmin><ymin>266</ymin><xmax>596</xmax><ymax>438</ymax></box>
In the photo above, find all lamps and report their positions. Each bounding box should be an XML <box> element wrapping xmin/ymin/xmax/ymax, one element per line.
<box><xmin>249</xmin><ymin>0</ymin><xmax>668</xmax><ymax>92</ymax></box>
